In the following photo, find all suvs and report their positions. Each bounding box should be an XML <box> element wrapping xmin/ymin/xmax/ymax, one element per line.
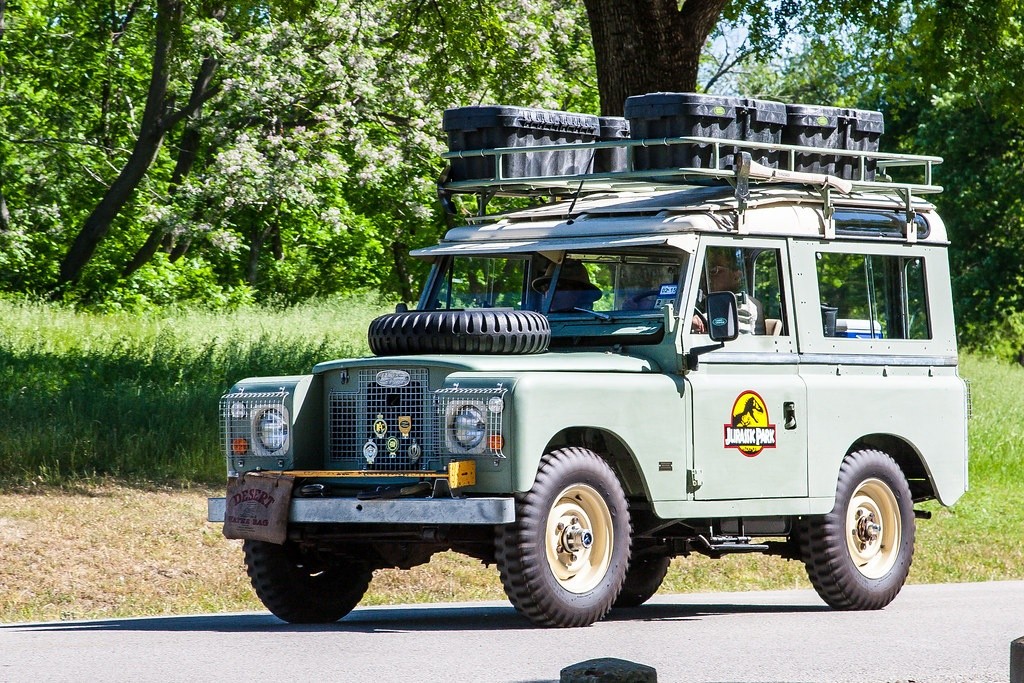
<box><xmin>205</xmin><ymin>93</ymin><xmax>972</xmax><ymax>626</ymax></box>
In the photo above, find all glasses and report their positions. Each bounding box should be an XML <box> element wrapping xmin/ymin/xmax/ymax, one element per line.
<box><xmin>709</xmin><ymin>264</ymin><xmax>731</xmax><ymax>275</ymax></box>
<box><xmin>539</xmin><ymin>281</ymin><xmax>581</xmax><ymax>292</ymax></box>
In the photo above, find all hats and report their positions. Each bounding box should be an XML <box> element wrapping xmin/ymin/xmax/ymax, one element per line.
<box><xmin>532</xmin><ymin>258</ymin><xmax>602</xmax><ymax>301</ymax></box>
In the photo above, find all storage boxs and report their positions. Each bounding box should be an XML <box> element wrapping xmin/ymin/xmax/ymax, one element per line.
<box><xmin>623</xmin><ymin>91</ymin><xmax>885</xmax><ymax>180</ymax></box>
<box><xmin>836</xmin><ymin>318</ymin><xmax>884</xmax><ymax>339</ymax></box>
<box><xmin>442</xmin><ymin>106</ymin><xmax>632</xmax><ymax>178</ymax></box>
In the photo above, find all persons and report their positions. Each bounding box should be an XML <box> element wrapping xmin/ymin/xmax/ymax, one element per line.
<box><xmin>529</xmin><ymin>259</ymin><xmax>602</xmax><ymax>314</ymax></box>
<box><xmin>692</xmin><ymin>246</ymin><xmax>765</xmax><ymax>335</ymax></box>
